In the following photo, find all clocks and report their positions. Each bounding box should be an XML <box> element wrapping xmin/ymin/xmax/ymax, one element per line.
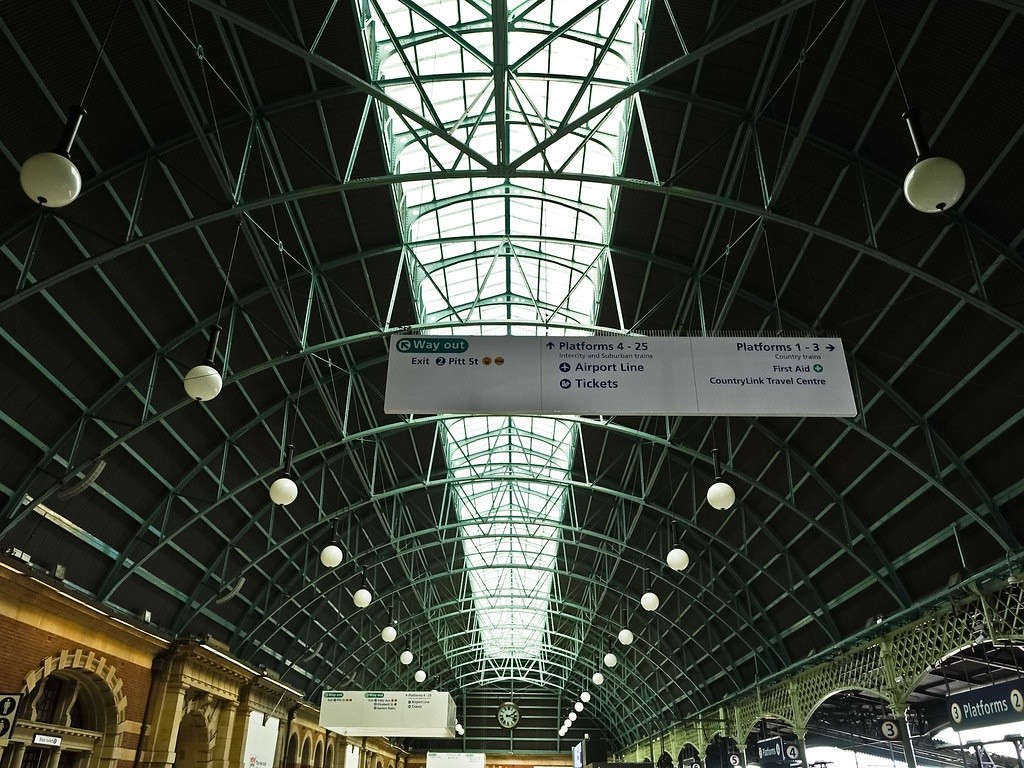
<box><xmin>496</xmin><ymin>701</ymin><xmax>522</xmax><ymax>731</ymax></box>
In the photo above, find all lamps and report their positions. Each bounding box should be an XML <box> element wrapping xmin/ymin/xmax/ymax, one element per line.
<box><xmin>138</xmin><ymin>610</ymin><xmax>152</xmax><ymax>625</ymax></box>
<box><xmin>640</xmin><ymin>568</ymin><xmax>660</xmax><ymax>612</ymax></box>
<box><xmin>666</xmin><ymin>519</ymin><xmax>689</xmax><ymax>572</ymax></box>
<box><xmin>48</xmin><ymin>564</ymin><xmax>66</xmax><ymax>581</ymax></box>
<box><xmin>431</xmin><ymin>676</ymin><xmax>465</xmax><ymax>735</ymax></box>
<box><xmin>20</xmin><ymin>105</ymin><xmax>88</xmax><ymax>209</ymax></box>
<box><xmin>320</xmin><ymin>517</ymin><xmax>343</xmax><ymax>569</ymax></box>
<box><xmin>382</xmin><ymin>605</ymin><xmax>397</xmax><ymax>643</ymax></box>
<box><xmin>270</xmin><ymin>443</ymin><xmax>297</xmax><ymax>506</ymax></box>
<box><xmin>705</xmin><ymin>448</ymin><xmax>736</xmax><ymax>513</ymax></box>
<box><xmin>806</xmin><ymin>648</ymin><xmax>817</xmax><ymax>658</ymax></box>
<box><xmin>863</xmin><ymin>617</ymin><xmax>876</xmax><ymax>628</ymax></box>
<box><xmin>184</xmin><ymin>324</ymin><xmax>222</xmax><ymax>403</ymax></box>
<box><xmin>414</xmin><ymin>655</ymin><xmax>426</xmax><ymax>683</ymax></box>
<box><xmin>558</xmin><ymin>689</ymin><xmax>583</xmax><ymax>737</ymax></box>
<box><xmin>400</xmin><ymin>633</ymin><xmax>413</xmax><ymax>664</ymax></box>
<box><xmin>353</xmin><ymin>565</ymin><xmax>373</xmax><ymax>609</ymax></box>
<box><xmin>604</xmin><ymin>634</ymin><xmax>617</xmax><ymax>667</ymax></box>
<box><xmin>947</xmin><ymin>571</ymin><xmax>963</xmax><ymax>588</ymax></box>
<box><xmin>903</xmin><ymin>107</ymin><xmax>966</xmax><ymax>214</ymax></box>
<box><xmin>580</xmin><ymin>678</ymin><xmax>591</xmax><ymax>702</ymax></box>
<box><xmin>618</xmin><ymin>609</ymin><xmax>634</xmax><ymax>646</ymax></box>
